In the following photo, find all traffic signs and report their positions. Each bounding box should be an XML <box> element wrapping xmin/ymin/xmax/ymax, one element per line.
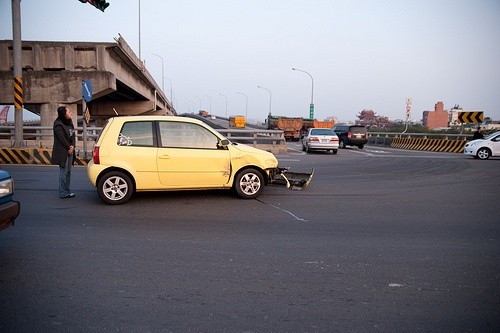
<box><xmin>457</xmin><ymin>112</ymin><xmax>484</xmax><ymax>124</ymax></box>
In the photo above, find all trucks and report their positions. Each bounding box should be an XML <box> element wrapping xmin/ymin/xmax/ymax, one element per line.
<box><xmin>303</xmin><ymin>117</ymin><xmax>335</xmax><ymax>136</ymax></box>
<box><xmin>199</xmin><ymin>110</ymin><xmax>208</xmax><ymax>118</ymax></box>
<box><xmin>228</xmin><ymin>115</ymin><xmax>246</xmax><ymax>128</ymax></box>
<box><xmin>265</xmin><ymin>114</ymin><xmax>302</xmax><ymax>142</ymax></box>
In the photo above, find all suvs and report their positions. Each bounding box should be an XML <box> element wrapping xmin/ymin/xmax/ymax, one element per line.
<box><xmin>332</xmin><ymin>124</ymin><xmax>368</xmax><ymax>150</ymax></box>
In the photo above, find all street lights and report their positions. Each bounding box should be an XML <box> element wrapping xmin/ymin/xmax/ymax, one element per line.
<box><xmin>257</xmin><ymin>86</ymin><xmax>271</xmax><ymax>115</ymax></box>
<box><xmin>151</xmin><ymin>53</ymin><xmax>166</xmax><ymax>94</ymax></box>
<box><xmin>205</xmin><ymin>95</ymin><xmax>211</xmax><ymax>116</ymax></box>
<box><xmin>236</xmin><ymin>91</ymin><xmax>248</xmax><ymax>119</ymax></box>
<box><xmin>218</xmin><ymin>93</ymin><xmax>227</xmax><ymax>119</ymax></box>
<box><xmin>166</xmin><ymin>78</ymin><xmax>172</xmax><ymax>106</ymax></box>
<box><xmin>292</xmin><ymin>68</ymin><xmax>313</xmax><ymax>119</ymax></box>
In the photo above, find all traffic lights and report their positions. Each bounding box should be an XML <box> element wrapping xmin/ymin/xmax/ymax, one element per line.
<box><xmin>78</xmin><ymin>0</ymin><xmax>109</xmax><ymax>13</ymax></box>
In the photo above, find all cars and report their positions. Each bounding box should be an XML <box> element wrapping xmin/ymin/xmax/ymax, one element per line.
<box><xmin>0</xmin><ymin>169</ymin><xmax>20</xmax><ymax>228</ymax></box>
<box><xmin>463</xmin><ymin>131</ymin><xmax>500</xmax><ymax>160</ymax></box>
<box><xmin>210</xmin><ymin>115</ymin><xmax>217</xmax><ymax>120</ymax></box>
<box><xmin>302</xmin><ymin>127</ymin><xmax>339</xmax><ymax>154</ymax></box>
<box><xmin>87</xmin><ymin>115</ymin><xmax>279</xmax><ymax>205</ymax></box>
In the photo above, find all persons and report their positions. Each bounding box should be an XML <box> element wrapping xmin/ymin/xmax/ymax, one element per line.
<box><xmin>51</xmin><ymin>106</ymin><xmax>76</xmax><ymax>199</ymax></box>
<box><xmin>473</xmin><ymin>127</ymin><xmax>485</xmax><ymax>140</ymax></box>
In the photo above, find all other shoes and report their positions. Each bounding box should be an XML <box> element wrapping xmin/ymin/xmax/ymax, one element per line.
<box><xmin>59</xmin><ymin>192</ymin><xmax>75</xmax><ymax>198</ymax></box>
